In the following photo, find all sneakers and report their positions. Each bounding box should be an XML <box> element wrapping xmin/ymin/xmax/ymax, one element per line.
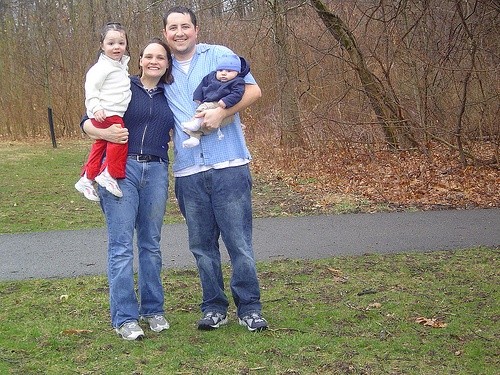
<box><xmin>141</xmin><ymin>315</ymin><xmax>169</xmax><ymax>333</ymax></box>
<box><xmin>239</xmin><ymin>312</ymin><xmax>268</xmax><ymax>332</ymax></box>
<box><xmin>95</xmin><ymin>172</ymin><xmax>124</xmax><ymax>197</ymax></box>
<box><xmin>116</xmin><ymin>321</ymin><xmax>145</xmax><ymax>340</ymax></box>
<box><xmin>198</xmin><ymin>312</ymin><xmax>228</xmax><ymax>330</ymax></box>
<box><xmin>74</xmin><ymin>177</ymin><xmax>101</xmax><ymax>201</ymax></box>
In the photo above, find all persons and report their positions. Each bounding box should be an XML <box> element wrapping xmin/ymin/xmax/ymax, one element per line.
<box><xmin>181</xmin><ymin>54</ymin><xmax>250</xmax><ymax>148</ymax></box>
<box><xmin>75</xmin><ymin>22</ymin><xmax>132</xmax><ymax>202</ymax></box>
<box><xmin>158</xmin><ymin>6</ymin><xmax>268</xmax><ymax>332</ymax></box>
<box><xmin>80</xmin><ymin>36</ymin><xmax>174</xmax><ymax>341</ymax></box>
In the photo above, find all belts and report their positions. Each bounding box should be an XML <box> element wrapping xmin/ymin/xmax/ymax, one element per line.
<box><xmin>127</xmin><ymin>155</ymin><xmax>165</xmax><ymax>163</ymax></box>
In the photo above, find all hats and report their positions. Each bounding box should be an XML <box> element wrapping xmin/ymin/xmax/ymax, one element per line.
<box><xmin>216</xmin><ymin>54</ymin><xmax>241</xmax><ymax>71</ymax></box>
